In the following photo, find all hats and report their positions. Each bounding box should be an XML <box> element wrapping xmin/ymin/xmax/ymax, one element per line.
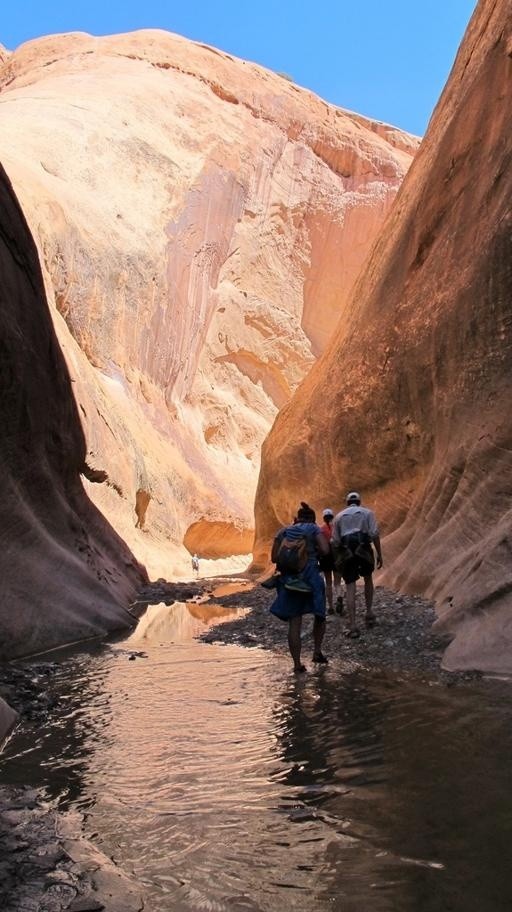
<box><xmin>346</xmin><ymin>492</ymin><xmax>360</xmax><ymax>502</ymax></box>
<box><xmin>322</xmin><ymin>509</ymin><xmax>333</xmax><ymax>517</ymax></box>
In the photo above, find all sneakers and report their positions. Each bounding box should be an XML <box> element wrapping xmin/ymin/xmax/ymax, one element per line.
<box><xmin>355</xmin><ymin>543</ymin><xmax>375</xmax><ymax>564</ymax></box>
<box><xmin>284</xmin><ymin>578</ymin><xmax>313</xmax><ymax>593</ymax></box>
<box><xmin>341</xmin><ymin>545</ymin><xmax>353</xmax><ymax>561</ymax></box>
<box><xmin>261</xmin><ymin>575</ymin><xmax>278</xmax><ymax>589</ymax></box>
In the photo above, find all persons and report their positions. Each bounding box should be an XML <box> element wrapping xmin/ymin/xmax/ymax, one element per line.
<box><xmin>192</xmin><ymin>553</ymin><xmax>199</xmax><ymax>579</ymax></box>
<box><xmin>270</xmin><ymin>501</ymin><xmax>330</xmax><ymax>676</ymax></box>
<box><xmin>318</xmin><ymin>506</ymin><xmax>345</xmax><ymax>617</ymax></box>
<box><xmin>330</xmin><ymin>491</ymin><xmax>384</xmax><ymax>636</ymax></box>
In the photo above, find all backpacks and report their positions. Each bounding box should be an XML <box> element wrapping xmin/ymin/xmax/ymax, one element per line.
<box><xmin>275</xmin><ymin>527</ymin><xmax>309</xmax><ymax>574</ymax></box>
